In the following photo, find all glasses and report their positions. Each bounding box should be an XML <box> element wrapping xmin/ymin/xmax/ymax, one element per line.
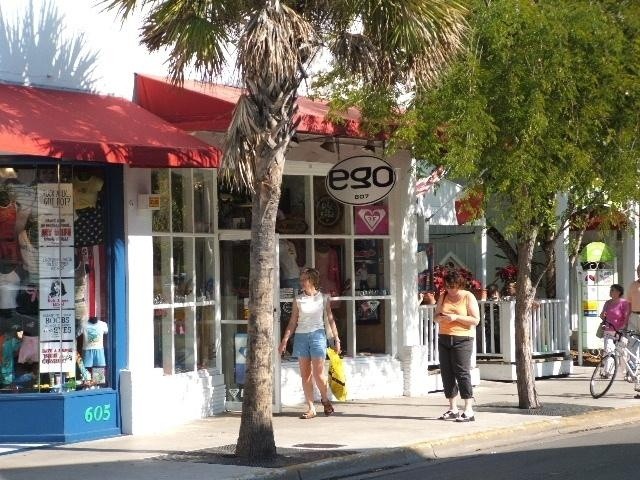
<box><xmin>446</xmin><ymin>285</ymin><xmax>456</xmax><ymax>289</ymax></box>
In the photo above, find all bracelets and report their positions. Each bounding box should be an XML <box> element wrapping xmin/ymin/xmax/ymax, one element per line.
<box><xmin>336</xmin><ymin>339</ymin><xmax>341</xmax><ymax>343</ymax></box>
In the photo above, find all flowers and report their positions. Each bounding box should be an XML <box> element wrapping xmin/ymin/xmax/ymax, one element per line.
<box><xmin>419</xmin><ymin>266</ymin><xmax>481</xmax><ymax>294</ymax></box>
<box><xmin>495</xmin><ymin>265</ymin><xmax>518</xmax><ymax>280</ymax></box>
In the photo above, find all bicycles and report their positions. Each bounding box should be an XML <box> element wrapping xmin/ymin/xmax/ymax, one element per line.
<box><xmin>590</xmin><ymin>320</ymin><xmax>640</xmax><ymax>398</ymax></box>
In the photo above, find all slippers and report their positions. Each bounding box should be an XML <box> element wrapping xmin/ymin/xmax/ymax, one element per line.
<box><xmin>438</xmin><ymin>410</ymin><xmax>475</xmax><ymax>422</ymax></box>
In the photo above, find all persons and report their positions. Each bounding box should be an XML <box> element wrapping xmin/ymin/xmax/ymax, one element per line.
<box><xmin>315</xmin><ymin>240</ymin><xmax>342</xmax><ymax>310</ymax></box>
<box><xmin>432</xmin><ymin>271</ymin><xmax>480</xmax><ymax>422</ymax></box>
<box><xmin>279</xmin><ymin>266</ymin><xmax>341</xmax><ymax>419</ymax></box>
<box><xmin>624</xmin><ymin>264</ymin><xmax>640</xmax><ymax>384</ymax></box>
<box><xmin>356</xmin><ymin>261</ymin><xmax>370</xmax><ymax>290</ymax></box>
<box><xmin>280</xmin><ymin>239</ymin><xmax>301</xmax><ymax>289</ymax></box>
<box><xmin>0</xmin><ymin>170</ymin><xmax>109</xmax><ymax>389</ymax></box>
<box><xmin>598</xmin><ymin>284</ymin><xmax>631</xmax><ymax>380</ymax></box>
<box><xmin>485</xmin><ymin>280</ymin><xmax>517</xmax><ymax>353</ymax></box>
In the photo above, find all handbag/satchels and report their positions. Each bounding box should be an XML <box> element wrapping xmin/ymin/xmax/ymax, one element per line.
<box><xmin>596</xmin><ymin>323</ymin><xmax>605</xmax><ymax>338</ymax></box>
<box><xmin>326</xmin><ymin>347</ymin><xmax>347</xmax><ymax>402</ymax></box>
<box><xmin>323</xmin><ymin>310</ymin><xmax>334</xmax><ymax>341</ymax></box>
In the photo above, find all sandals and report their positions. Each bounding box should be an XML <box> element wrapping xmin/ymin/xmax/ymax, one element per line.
<box><xmin>321</xmin><ymin>399</ymin><xmax>334</xmax><ymax>416</ymax></box>
<box><xmin>300</xmin><ymin>409</ymin><xmax>317</xmax><ymax>419</ymax></box>
